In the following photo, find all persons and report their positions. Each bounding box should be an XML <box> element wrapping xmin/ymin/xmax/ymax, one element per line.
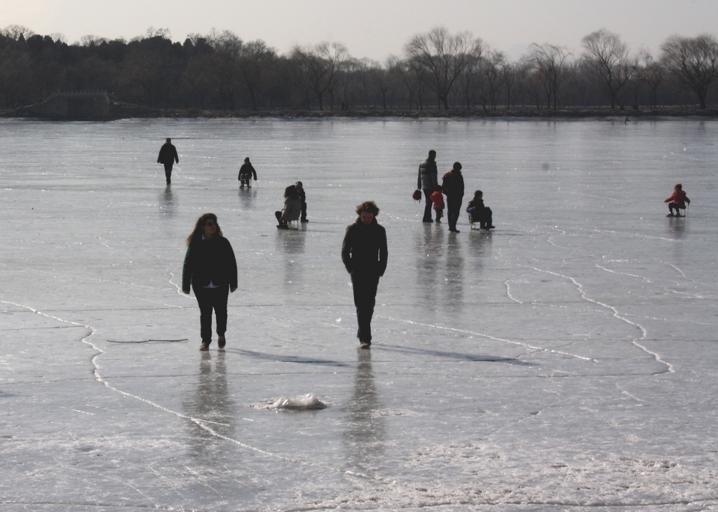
<box><xmin>341</xmin><ymin>201</ymin><xmax>388</xmax><ymax>350</ymax></box>
<box><xmin>417</xmin><ymin>149</ymin><xmax>437</xmax><ymax>223</ymax></box>
<box><xmin>180</xmin><ymin>212</ymin><xmax>238</xmax><ymax>351</ymax></box>
<box><xmin>157</xmin><ymin>139</ymin><xmax>178</xmax><ymax>186</ymax></box>
<box><xmin>443</xmin><ymin>162</ymin><xmax>464</xmax><ymax>233</ymax></box>
<box><xmin>296</xmin><ymin>181</ymin><xmax>310</xmax><ymax>224</ymax></box>
<box><xmin>237</xmin><ymin>156</ymin><xmax>256</xmax><ymax>189</ymax></box>
<box><xmin>271</xmin><ymin>185</ymin><xmax>300</xmax><ymax>229</ymax></box>
<box><xmin>663</xmin><ymin>184</ymin><xmax>691</xmax><ymax>217</ymax></box>
<box><xmin>467</xmin><ymin>189</ymin><xmax>496</xmax><ymax>233</ymax></box>
<box><xmin>429</xmin><ymin>185</ymin><xmax>445</xmax><ymax>223</ymax></box>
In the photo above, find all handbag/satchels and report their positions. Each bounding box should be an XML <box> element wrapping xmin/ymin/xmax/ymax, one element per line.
<box><xmin>413</xmin><ymin>189</ymin><xmax>421</xmax><ymax>200</ymax></box>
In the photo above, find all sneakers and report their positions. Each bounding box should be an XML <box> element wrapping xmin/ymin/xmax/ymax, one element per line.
<box><xmin>200</xmin><ymin>342</ymin><xmax>209</xmax><ymax>351</ymax></box>
<box><xmin>218</xmin><ymin>335</ymin><xmax>225</xmax><ymax>348</ymax></box>
<box><xmin>360</xmin><ymin>342</ymin><xmax>370</xmax><ymax>349</ymax></box>
